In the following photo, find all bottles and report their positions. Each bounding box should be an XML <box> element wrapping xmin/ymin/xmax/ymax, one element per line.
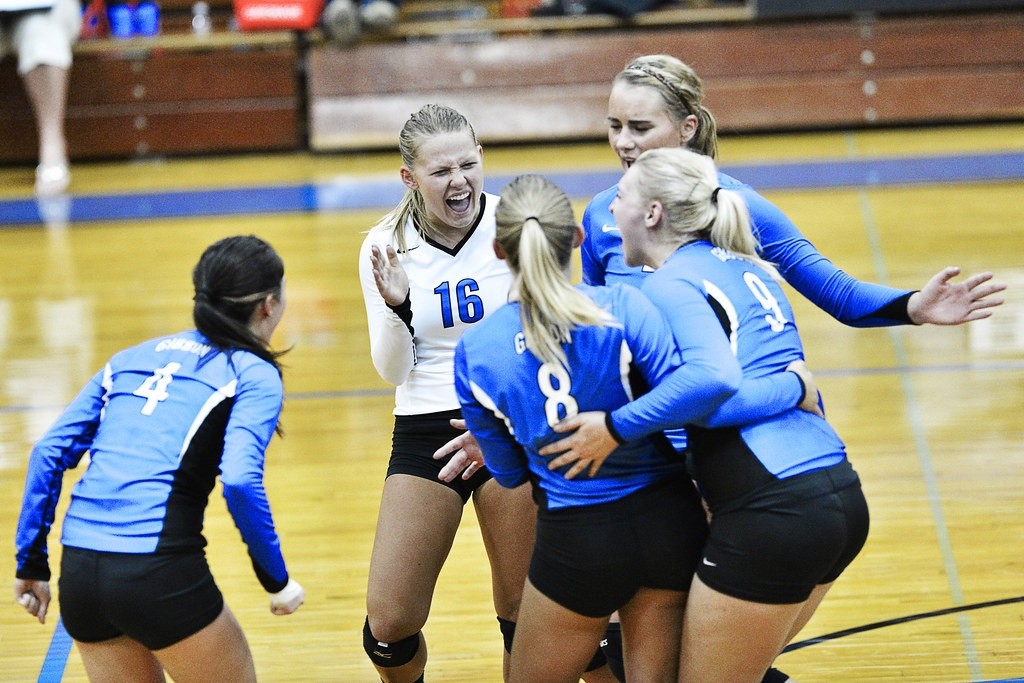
<box><xmin>192</xmin><ymin>1</ymin><xmax>212</xmax><ymax>42</ymax></box>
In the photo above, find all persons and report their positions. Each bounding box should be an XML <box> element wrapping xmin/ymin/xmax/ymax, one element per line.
<box><xmin>12</xmin><ymin>232</ymin><xmax>309</xmax><ymax>682</ymax></box>
<box><xmin>17</xmin><ymin>0</ymin><xmax>85</xmax><ymax>198</ymax></box>
<box><xmin>357</xmin><ymin>52</ymin><xmax>1007</xmax><ymax>682</ymax></box>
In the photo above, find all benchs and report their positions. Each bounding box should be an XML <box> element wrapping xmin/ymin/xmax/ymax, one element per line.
<box><xmin>72</xmin><ymin>0</ymin><xmax>761</xmax><ymax>53</ymax></box>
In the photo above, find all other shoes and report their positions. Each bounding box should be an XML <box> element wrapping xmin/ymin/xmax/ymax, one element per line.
<box><xmin>33</xmin><ymin>158</ymin><xmax>70</xmax><ymax>198</ymax></box>
